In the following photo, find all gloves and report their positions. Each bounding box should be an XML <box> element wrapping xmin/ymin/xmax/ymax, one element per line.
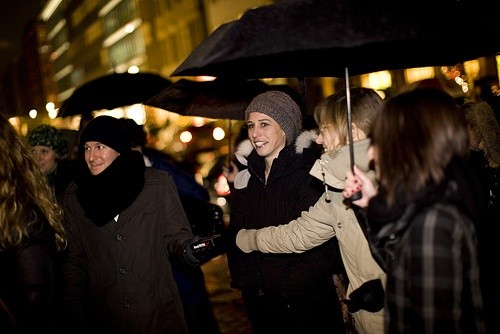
<box><xmin>225</xmin><ymin>230</ymin><xmax>238</xmax><ymax>251</ymax></box>
<box><xmin>349</xmin><ymin>279</ymin><xmax>385</xmax><ymax>313</ymax></box>
<box><xmin>191</xmin><ymin>234</ymin><xmax>223</xmax><ymax>262</ymax></box>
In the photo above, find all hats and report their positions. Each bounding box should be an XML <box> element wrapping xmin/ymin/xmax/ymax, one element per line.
<box><xmin>28</xmin><ymin>124</ymin><xmax>65</xmax><ymax>153</ymax></box>
<box><xmin>245</xmin><ymin>91</ymin><xmax>302</xmax><ymax>146</ymax></box>
<box><xmin>80</xmin><ymin>115</ymin><xmax>130</xmax><ymax>155</ymax></box>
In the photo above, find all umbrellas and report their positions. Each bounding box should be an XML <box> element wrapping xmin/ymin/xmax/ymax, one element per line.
<box><xmin>57</xmin><ymin>0</ymin><xmax>500</xmax><ymax>179</ymax></box>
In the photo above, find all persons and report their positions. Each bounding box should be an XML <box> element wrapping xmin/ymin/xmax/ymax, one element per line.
<box><xmin>1</xmin><ymin>114</ymin><xmax>70</xmax><ymax>334</ymax></box>
<box><xmin>30</xmin><ymin>75</ymin><xmax>500</xmax><ymax>334</ymax></box>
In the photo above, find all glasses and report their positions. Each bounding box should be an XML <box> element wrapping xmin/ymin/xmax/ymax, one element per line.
<box><xmin>317</xmin><ymin>126</ymin><xmax>334</xmax><ymax>135</ymax></box>
